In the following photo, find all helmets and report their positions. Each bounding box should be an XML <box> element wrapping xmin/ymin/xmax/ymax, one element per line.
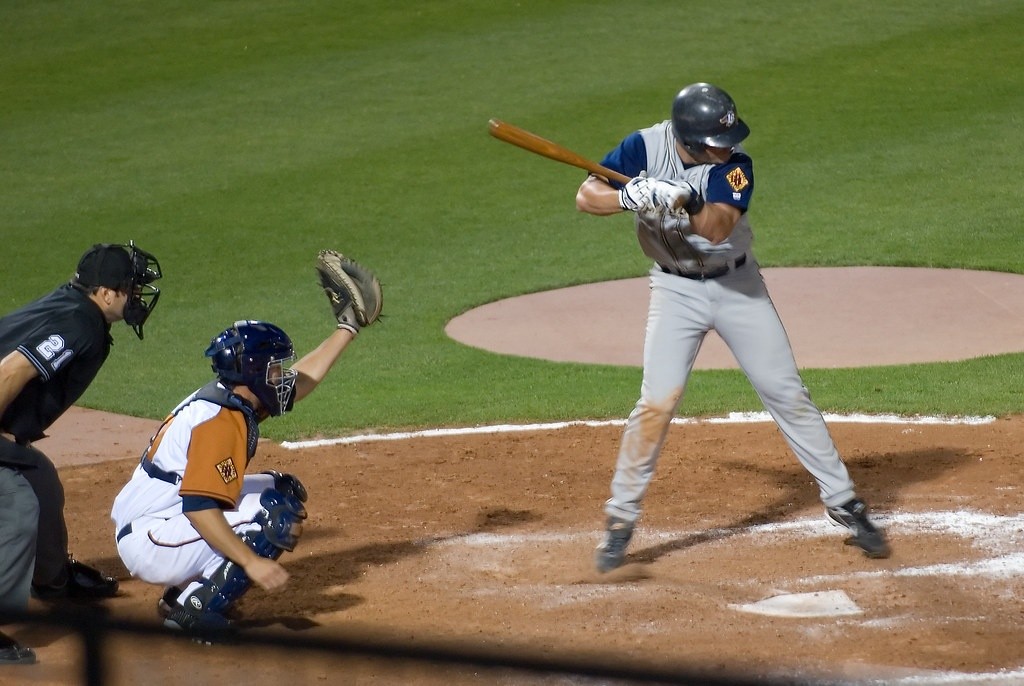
<box><xmin>204</xmin><ymin>321</ymin><xmax>297</xmax><ymax>418</ymax></box>
<box><xmin>671</xmin><ymin>82</ymin><xmax>750</xmax><ymax>155</ymax></box>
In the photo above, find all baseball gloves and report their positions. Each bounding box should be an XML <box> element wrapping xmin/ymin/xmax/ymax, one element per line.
<box><xmin>313</xmin><ymin>245</ymin><xmax>388</xmax><ymax>336</ymax></box>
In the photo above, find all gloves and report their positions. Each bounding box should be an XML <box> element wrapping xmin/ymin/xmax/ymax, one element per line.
<box><xmin>654</xmin><ymin>179</ymin><xmax>698</xmax><ymax>215</ymax></box>
<box><xmin>619</xmin><ymin>171</ymin><xmax>655</xmax><ymax>211</ymax></box>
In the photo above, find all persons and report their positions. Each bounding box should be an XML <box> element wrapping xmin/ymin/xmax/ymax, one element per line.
<box><xmin>0</xmin><ymin>243</ymin><xmax>164</xmax><ymax>664</ymax></box>
<box><xmin>576</xmin><ymin>83</ymin><xmax>890</xmax><ymax>573</ymax></box>
<box><xmin>111</xmin><ymin>249</ymin><xmax>383</xmax><ymax>644</ymax></box>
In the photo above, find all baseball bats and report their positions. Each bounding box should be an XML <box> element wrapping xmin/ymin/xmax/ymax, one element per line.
<box><xmin>487</xmin><ymin>117</ymin><xmax>687</xmax><ymax>210</ymax></box>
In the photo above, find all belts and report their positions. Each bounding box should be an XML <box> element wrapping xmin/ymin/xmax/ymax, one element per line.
<box><xmin>662</xmin><ymin>252</ymin><xmax>747</xmax><ymax>281</ymax></box>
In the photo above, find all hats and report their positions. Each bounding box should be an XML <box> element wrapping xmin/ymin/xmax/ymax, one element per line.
<box><xmin>74</xmin><ymin>243</ymin><xmax>157</xmax><ymax>291</ymax></box>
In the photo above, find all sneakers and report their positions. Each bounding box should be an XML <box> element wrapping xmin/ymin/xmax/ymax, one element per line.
<box><xmin>159</xmin><ymin>586</ymin><xmax>182</xmax><ymax>612</ymax></box>
<box><xmin>0</xmin><ymin>634</ymin><xmax>36</xmax><ymax>664</ymax></box>
<box><xmin>824</xmin><ymin>498</ymin><xmax>888</xmax><ymax>558</ymax></box>
<box><xmin>163</xmin><ymin>603</ymin><xmax>238</xmax><ymax>631</ymax></box>
<box><xmin>30</xmin><ymin>558</ymin><xmax>119</xmax><ymax>600</ymax></box>
<box><xmin>594</xmin><ymin>516</ymin><xmax>635</xmax><ymax>572</ymax></box>
